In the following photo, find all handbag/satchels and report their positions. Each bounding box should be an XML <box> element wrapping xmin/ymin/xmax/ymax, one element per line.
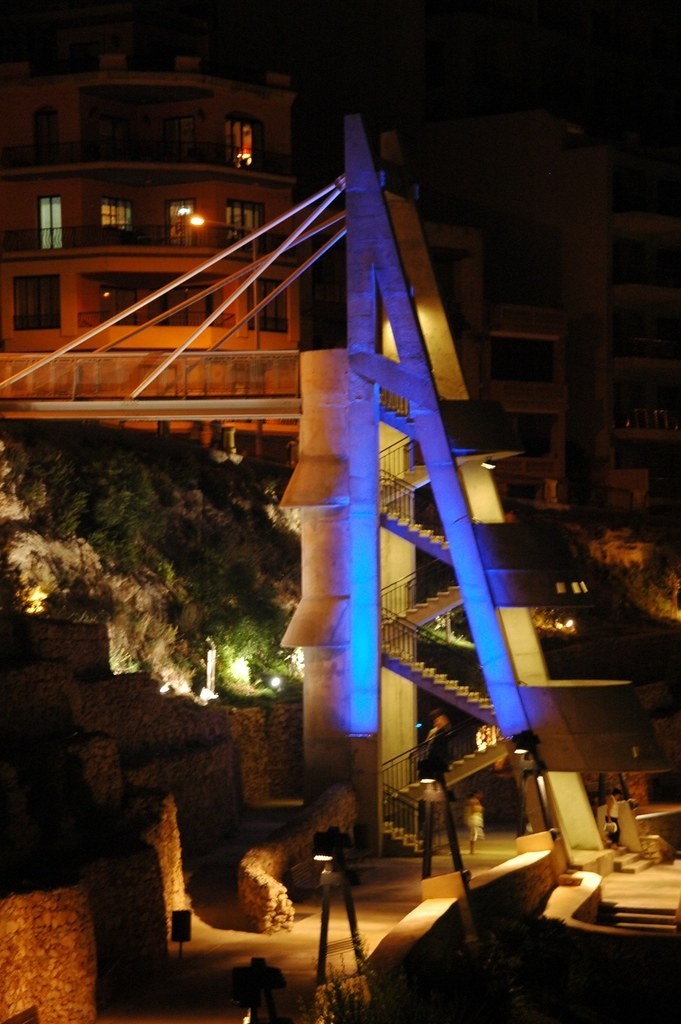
<box><xmin>604</xmin><ymin>820</ymin><xmax>617</xmax><ymax>833</ymax></box>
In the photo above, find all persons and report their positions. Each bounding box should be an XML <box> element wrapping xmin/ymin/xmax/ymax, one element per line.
<box><xmin>463</xmin><ymin>788</ymin><xmax>484</xmax><ymax>854</ymax></box>
<box><xmin>600</xmin><ymin>788</ymin><xmax>621</xmax><ymax>850</ymax></box>
<box><xmin>415</xmin><ymin>705</ymin><xmax>452</xmax><ymax>788</ymax></box>
